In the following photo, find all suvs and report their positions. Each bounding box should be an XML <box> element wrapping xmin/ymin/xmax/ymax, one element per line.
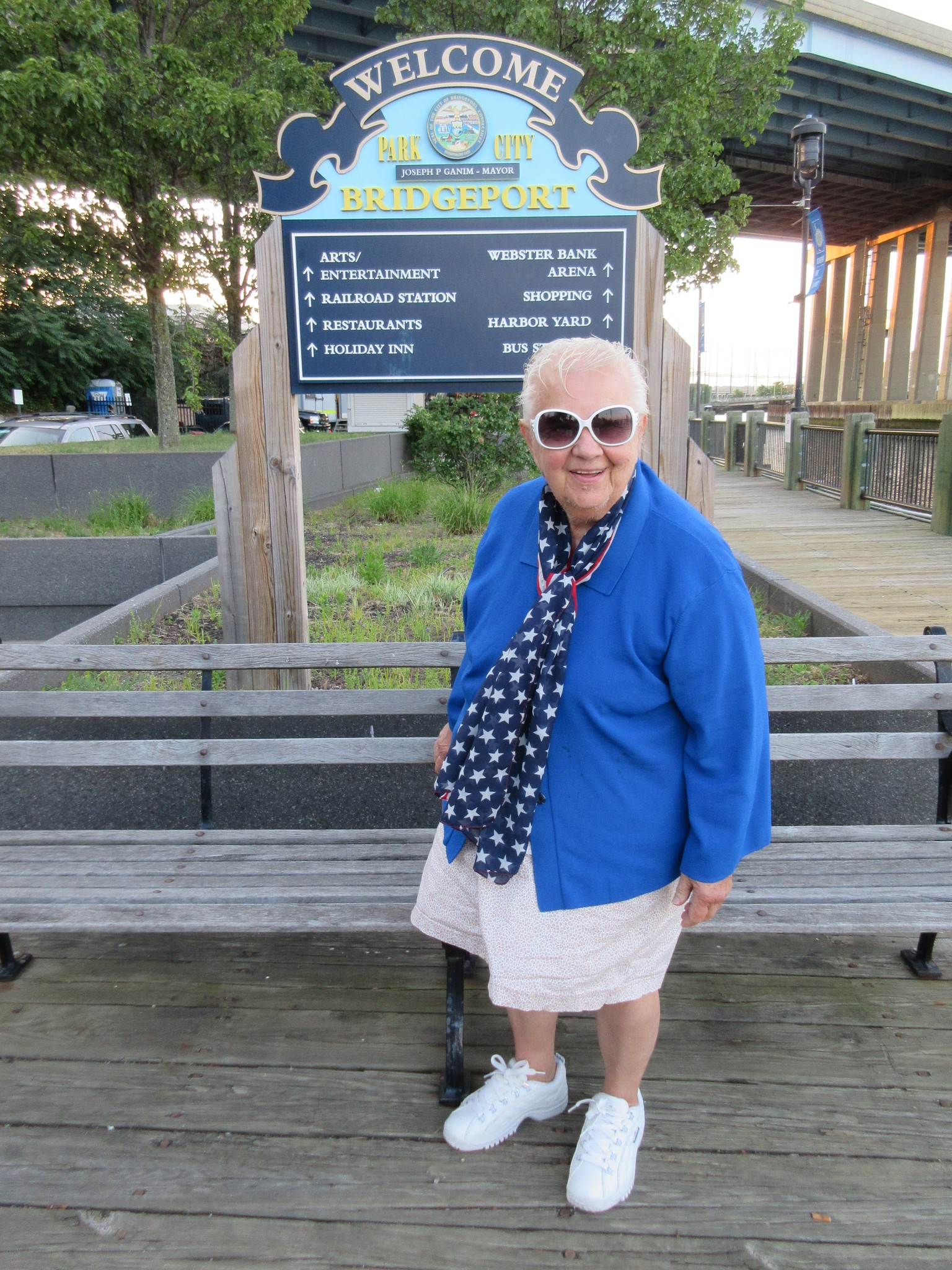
<box><xmin>4</xmin><ymin>398</ymin><xmax>337</xmax><ymax>442</ymax></box>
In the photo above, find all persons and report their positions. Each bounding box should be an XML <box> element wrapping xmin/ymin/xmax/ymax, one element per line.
<box><xmin>406</xmin><ymin>338</ymin><xmax>773</xmax><ymax>1216</ymax></box>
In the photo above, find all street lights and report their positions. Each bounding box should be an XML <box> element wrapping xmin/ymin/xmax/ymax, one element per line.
<box><xmin>789</xmin><ymin>109</ymin><xmax>825</xmax><ymax>416</ymax></box>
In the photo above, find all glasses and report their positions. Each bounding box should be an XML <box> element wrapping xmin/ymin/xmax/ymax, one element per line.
<box><xmin>523</xmin><ymin>403</ymin><xmax>644</xmax><ymax>451</ymax></box>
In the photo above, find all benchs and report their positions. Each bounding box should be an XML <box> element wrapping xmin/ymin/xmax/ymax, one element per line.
<box><xmin>0</xmin><ymin>638</ymin><xmax>491</xmax><ymax>1110</ymax></box>
<box><xmin>678</xmin><ymin>629</ymin><xmax>952</xmax><ymax>983</ymax></box>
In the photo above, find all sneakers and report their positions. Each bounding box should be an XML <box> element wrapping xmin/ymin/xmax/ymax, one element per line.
<box><xmin>566</xmin><ymin>1088</ymin><xmax>646</xmax><ymax>1213</ymax></box>
<box><xmin>443</xmin><ymin>1052</ymin><xmax>567</xmax><ymax>1152</ymax></box>
